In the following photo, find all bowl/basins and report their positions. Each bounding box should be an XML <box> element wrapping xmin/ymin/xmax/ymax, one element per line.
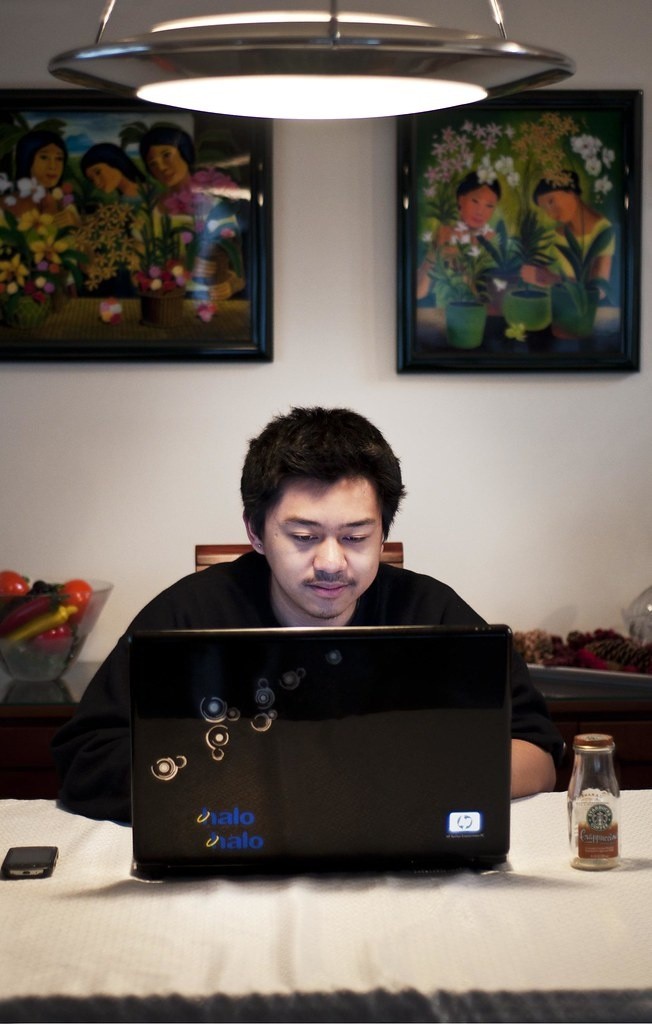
<box><xmin>0</xmin><ymin>582</ymin><xmax>112</xmax><ymax>681</ymax></box>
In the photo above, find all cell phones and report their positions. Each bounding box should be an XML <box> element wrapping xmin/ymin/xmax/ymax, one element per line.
<box><xmin>1</xmin><ymin>847</ymin><xmax>59</xmax><ymax>880</ymax></box>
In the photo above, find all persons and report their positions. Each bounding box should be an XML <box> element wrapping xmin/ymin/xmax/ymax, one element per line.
<box><xmin>50</xmin><ymin>406</ymin><xmax>569</xmax><ymax>826</ymax></box>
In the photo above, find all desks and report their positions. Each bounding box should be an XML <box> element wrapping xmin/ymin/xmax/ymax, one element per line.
<box><xmin>0</xmin><ymin>790</ymin><xmax>652</xmax><ymax>1024</ymax></box>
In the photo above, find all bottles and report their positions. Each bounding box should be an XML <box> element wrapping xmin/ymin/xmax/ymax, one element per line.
<box><xmin>568</xmin><ymin>735</ymin><xmax>620</xmax><ymax>871</ymax></box>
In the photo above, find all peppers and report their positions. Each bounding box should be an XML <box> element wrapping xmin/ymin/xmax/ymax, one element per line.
<box><xmin>0</xmin><ymin>580</ymin><xmax>80</xmax><ymax>649</ymax></box>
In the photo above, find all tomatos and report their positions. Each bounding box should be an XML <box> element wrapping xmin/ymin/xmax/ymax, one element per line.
<box><xmin>0</xmin><ymin>571</ymin><xmax>95</xmax><ymax>624</ymax></box>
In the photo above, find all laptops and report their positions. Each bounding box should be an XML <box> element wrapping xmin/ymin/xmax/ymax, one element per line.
<box><xmin>125</xmin><ymin>625</ymin><xmax>515</xmax><ymax>874</ymax></box>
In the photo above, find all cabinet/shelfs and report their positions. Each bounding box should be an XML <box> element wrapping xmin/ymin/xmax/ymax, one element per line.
<box><xmin>0</xmin><ymin>658</ymin><xmax>652</xmax><ymax>798</ymax></box>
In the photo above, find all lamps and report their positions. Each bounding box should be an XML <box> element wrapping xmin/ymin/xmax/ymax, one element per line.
<box><xmin>46</xmin><ymin>0</ymin><xmax>576</xmax><ymax>120</ymax></box>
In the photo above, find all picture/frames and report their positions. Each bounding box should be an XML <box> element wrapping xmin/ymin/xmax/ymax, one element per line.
<box><xmin>0</xmin><ymin>87</ymin><xmax>275</xmax><ymax>364</ymax></box>
<box><xmin>396</xmin><ymin>88</ymin><xmax>644</xmax><ymax>376</ymax></box>
